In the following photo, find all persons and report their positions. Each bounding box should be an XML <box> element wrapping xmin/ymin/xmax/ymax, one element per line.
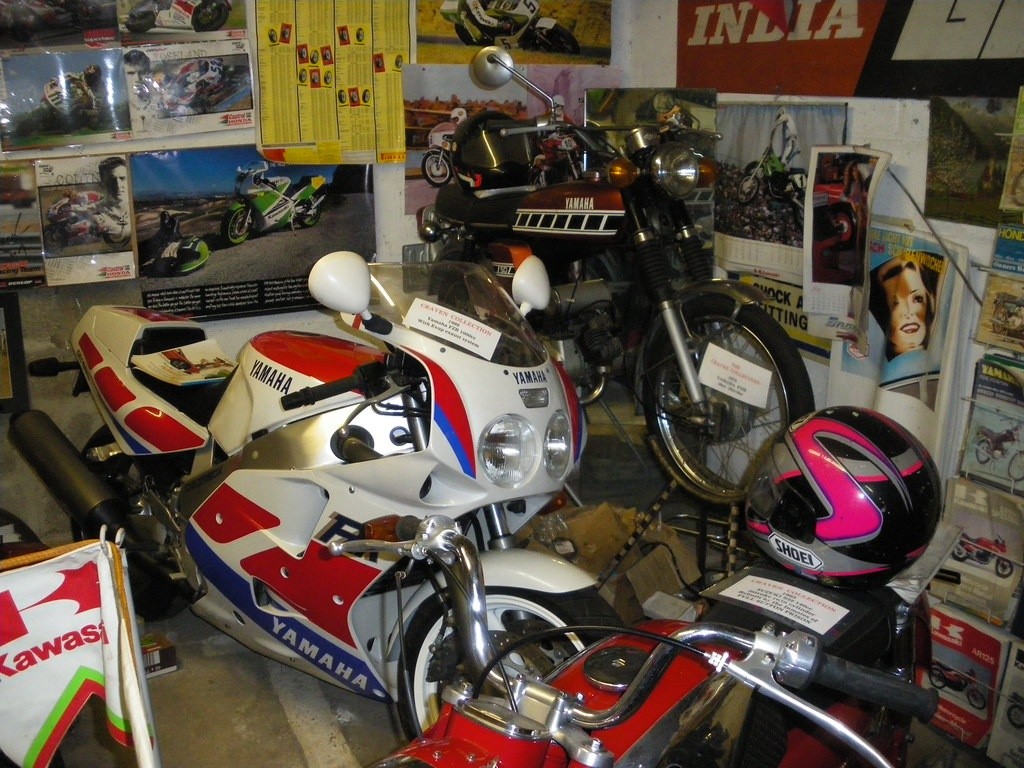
<box><xmin>99</xmin><ymin>157</ymin><xmax>132</xmax><ymax>229</ymax></box>
<box><xmin>544</xmin><ymin>95</ymin><xmax>576</xmax><ymax>149</ymax></box>
<box><xmin>58</xmin><ymin>63</ymin><xmax>108</xmax><ymax>112</ymax></box>
<box><xmin>125</xmin><ymin>50</ymin><xmax>192</xmax><ymax>118</ymax></box>
<box><xmin>452</xmin><ymin>107</ymin><xmax>467</xmax><ymax>125</ymax></box>
<box><xmin>773</xmin><ymin>106</ymin><xmax>802</xmax><ymax>166</ymax></box>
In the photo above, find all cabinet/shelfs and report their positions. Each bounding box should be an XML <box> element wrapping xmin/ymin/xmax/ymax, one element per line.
<box><xmin>914</xmin><ymin>85</ymin><xmax>1024</xmax><ymax>767</ymax></box>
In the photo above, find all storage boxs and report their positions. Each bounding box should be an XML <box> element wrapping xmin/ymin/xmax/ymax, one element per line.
<box><xmin>135</xmin><ymin>613</ymin><xmax>145</xmax><ymax>635</ymax></box>
<box><xmin>140</xmin><ymin>630</ymin><xmax>178</xmax><ymax>679</ymax></box>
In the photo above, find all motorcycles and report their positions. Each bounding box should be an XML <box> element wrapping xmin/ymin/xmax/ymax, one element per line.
<box><xmin>8</xmin><ymin>247</ymin><xmax>639</xmax><ymax>746</ymax></box>
<box><xmin>216</xmin><ymin>160</ymin><xmax>330</xmax><ymax>246</ymax></box>
<box><xmin>317</xmin><ymin>406</ymin><xmax>945</xmax><ymax>768</ymax></box>
<box><xmin>43</xmin><ymin>189</ymin><xmax>105</xmax><ymax>253</ymax></box>
<box><xmin>740</xmin><ymin>125</ymin><xmax>809</xmax><ymax>214</ymax></box>
<box><xmin>419</xmin><ymin>120</ymin><xmax>456</xmax><ymax>187</ymax></box>
<box><xmin>407</xmin><ymin>44</ymin><xmax>808</xmax><ymax>517</ymax></box>
<box><xmin>438</xmin><ymin>0</ymin><xmax>582</xmax><ymax>57</ymax></box>
<box><xmin>121</xmin><ymin>0</ymin><xmax>234</xmax><ymax>33</ymax></box>
<box><xmin>14</xmin><ymin>74</ymin><xmax>94</xmax><ymax>135</ymax></box>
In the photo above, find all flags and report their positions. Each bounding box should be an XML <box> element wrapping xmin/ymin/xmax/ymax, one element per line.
<box><xmin>0</xmin><ymin>542</ymin><xmax>157</xmax><ymax>767</ymax></box>
